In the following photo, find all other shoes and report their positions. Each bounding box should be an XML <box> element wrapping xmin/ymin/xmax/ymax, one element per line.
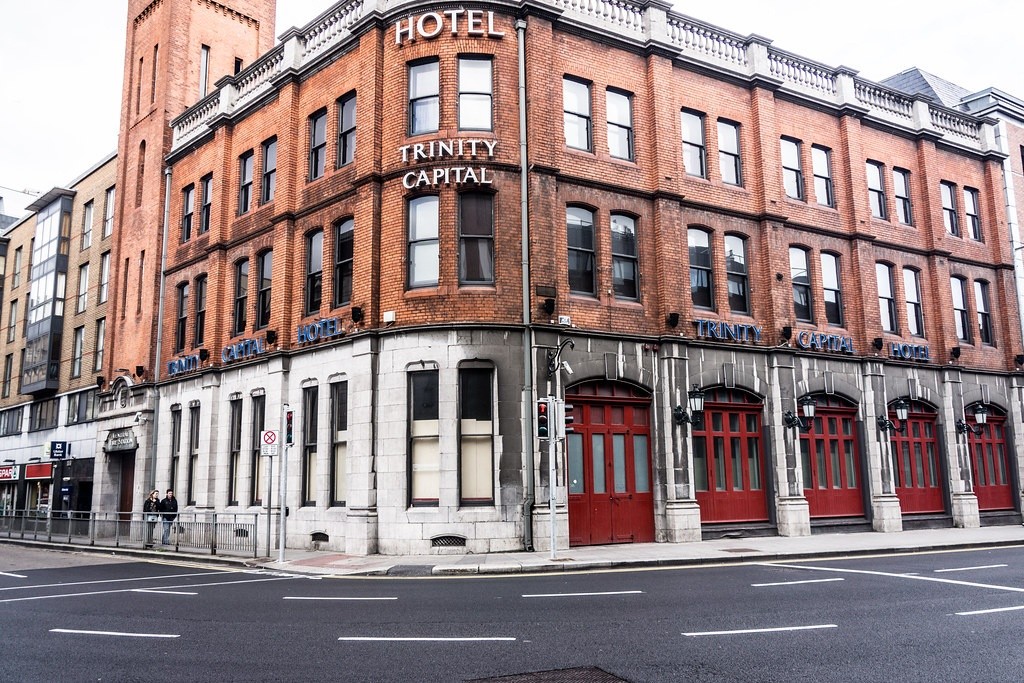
<box><xmin>146</xmin><ymin>545</ymin><xmax>153</xmax><ymax>548</ymax></box>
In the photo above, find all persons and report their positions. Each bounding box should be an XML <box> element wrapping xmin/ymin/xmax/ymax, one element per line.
<box><xmin>142</xmin><ymin>490</ymin><xmax>161</xmax><ymax>546</ymax></box>
<box><xmin>161</xmin><ymin>489</ymin><xmax>178</xmax><ymax>545</ymax></box>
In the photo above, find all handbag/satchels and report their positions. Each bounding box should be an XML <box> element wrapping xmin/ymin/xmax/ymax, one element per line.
<box><xmin>148</xmin><ymin>516</ymin><xmax>158</xmax><ymax>523</ymax></box>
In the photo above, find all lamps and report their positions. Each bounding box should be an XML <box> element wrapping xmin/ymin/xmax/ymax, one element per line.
<box><xmin>874</xmin><ymin>338</ymin><xmax>882</xmax><ymax>351</ymax></box>
<box><xmin>953</xmin><ymin>347</ymin><xmax>961</xmax><ymax>360</ymax></box>
<box><xmin>136</xmin><ymin>366</ymin><xmax>144</xmax><ymax>377</ymax></box>
<box><xmin>669</xmin><ymin>312</ymin><xmax>679</xmax><ymax>329</ymax></box>
<box><xmin>544</xmin><ymin>298</ymin><xmax>555</xmax><ymax>316</ymax></box>
<box><xmin>878</xmin><ymin>398</ymin><xmax>909</xmax><ymax>433</ymax></box>
<box><xmin>200</xmin><ymin>349</ymin><xmax>209</xmax><ymax>369</ymax></box>
<box><xmin>673</xmin><ymin>383</ymin><xmax>705</xmax><ymax>427</ymax></box>
<box><xmin>351</xmin><ymin>306</ymin><xmax>361</xmax><ymax>323</ymax></box>
<box><xmin>1016</xmin><ymin>354</ymin><xmax>1023</xmax><ymax>367</ymax></box>
<box><xmin>266</xmin><ymin>330</ymin><xmax>276</xmax><ymax>351</ymax></box>
<box><xmin>957</xmin><ymin>401</ymin><xmax>988</xmax><ymax>435</ymax></box>
<box><xmin>96</xmin><ymin>376</ymin><xmax>104</xmax><ymax>393</ymax></box>
<box><xmin>783</xmin><ymin>326</ymin><xmax>792</xmax><ymax>340</ymax></box>
<box><xmin>784</xmin><ymin>395</ymin><xmax>816</xmax><ymax>431</ymax></box>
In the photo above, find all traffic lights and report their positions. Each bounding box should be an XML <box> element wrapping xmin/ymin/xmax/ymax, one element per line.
<box><xmin>556</xmin><ymin>401</ymin><xmax>574</xmax><ymax>440</ymax></box>
<box><xmin>536</xmin><ymin>398</ymin><xmax>550</xmax><ymax>440</ymax></box>
